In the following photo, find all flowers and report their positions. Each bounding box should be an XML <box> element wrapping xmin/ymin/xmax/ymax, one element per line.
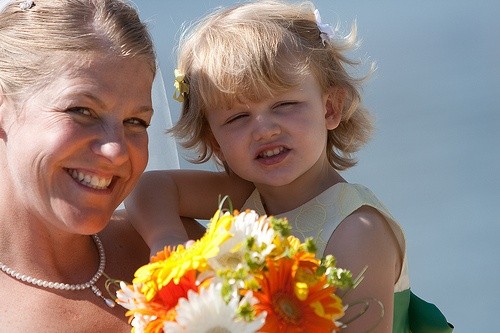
<box><xmin>114</xmin><ymin>194</ymin><xmax>385</xmax><ymax>333</ymax></box>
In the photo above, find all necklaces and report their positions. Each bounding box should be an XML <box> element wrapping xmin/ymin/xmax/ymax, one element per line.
<box><xmin>0</xmin><ymin>231</ymin><xmax>117</xmax><ymax>309</ymax></box>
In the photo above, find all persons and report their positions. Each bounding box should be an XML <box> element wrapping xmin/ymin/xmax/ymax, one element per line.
<box><xmin>1</xmin><ymin>0</ymin><xmax>211</xmax><ymax>333</ymax></box>
<box><xmin>121</xmin><ymin>0</ymin><xmax>454</xmax><ymax>333</ymax></box>
